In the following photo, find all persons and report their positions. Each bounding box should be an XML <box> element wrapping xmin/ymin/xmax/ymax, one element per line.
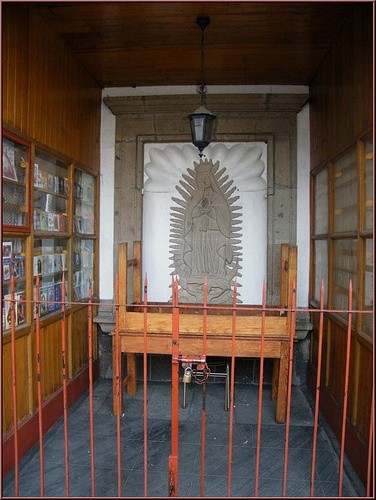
<box><xmin>180</xmin><ymin>163</ymin><xmax>232</xmax><ymax>279</ymax></box>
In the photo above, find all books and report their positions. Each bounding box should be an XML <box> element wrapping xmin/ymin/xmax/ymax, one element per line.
<box><xmin>3</xmin><ymin>137</ymin><xmax>96</xmax><ymax>328</ymax></box>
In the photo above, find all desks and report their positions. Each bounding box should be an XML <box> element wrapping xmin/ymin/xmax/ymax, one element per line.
<box><xmin>111</xmin><ymin>237</ymin><xmax>302</xmax><ymax>426</ymax></box>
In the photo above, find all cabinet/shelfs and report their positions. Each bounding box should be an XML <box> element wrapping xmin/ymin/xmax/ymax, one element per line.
<box><xmin>309</xmin><ymin>127</ymin><xmax>373</xmax><ymax>452</ymax></box>
<box><xmin>1</xmin><ymin>125</ymin><xmax>99</xmax><ymax>446</ymax></box>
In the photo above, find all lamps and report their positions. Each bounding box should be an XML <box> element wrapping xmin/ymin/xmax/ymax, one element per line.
<box><xmin>187</xmin><ymin>14</ymin><xmax>218</xmax><ymax>158</ymax></box>
